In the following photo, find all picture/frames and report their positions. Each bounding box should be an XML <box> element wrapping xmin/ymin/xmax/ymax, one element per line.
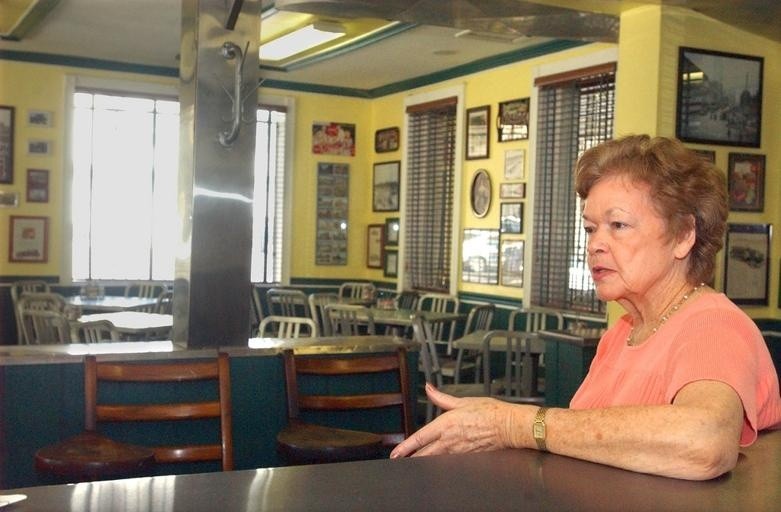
<box><xmin>25</xmin><ymin>138</ymin><xmax>49</xmax><ymax>155</ymax></box>
<box><xmin>676</xmin><ymin>45</ymin><xmax>770</xmax><ymax>305</ymax></box>
<box><xmin>26</xmin><ymin>108</ymin><xmax>51</xmax><ymax>128</ymax></box>
<box><xmin>26</xmin><ymin>167</ymin><xmax>48</xmax><ymax>201</ymax></box>
<box><xmin>459</xmin><ymin>98</ymin><xmax>530</xmax><ymax>289</ymax></box>
<box><xmin>375</xmin><ymin>126</ymin><xmax>399</xmax><ymax>153</ymax></box>
<box><xmin>8</xmin><ymin>215</ymin><xmax>49</xmax><ymax>264</ymax></box>
<box><xmin>367</xmin><ymin>218</ymin><xmax>399</xmax><ymax>279</ymax></box>
<box><xmin>0</xmin><ymin>188</ymin><xmax>20</xmax><ymax>208</ymax></box>
<box><xmin>0</xmin><ymin>105</ymin><xmax>15</xmax><ymax>183</ymax></box>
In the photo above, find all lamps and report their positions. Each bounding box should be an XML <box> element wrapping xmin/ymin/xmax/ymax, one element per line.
<box><xmin>256</xmin><ymin>20</ymin><xmax>346</xmax><ymax>63</ymax></box>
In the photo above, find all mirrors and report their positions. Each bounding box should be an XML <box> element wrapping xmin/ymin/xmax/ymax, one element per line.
<box><xmin>372</xmin><ymin>161</ymin><xmax>400</xmax><ymax>212</ymax></box>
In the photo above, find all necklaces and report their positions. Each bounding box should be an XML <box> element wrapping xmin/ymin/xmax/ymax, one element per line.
<box><xmin>624</xmin><ymin>282</ymin><xmax>705</xmax><ymax>347</ymax></box>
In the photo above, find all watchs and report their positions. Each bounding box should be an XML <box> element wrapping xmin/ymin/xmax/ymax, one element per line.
<box><xmin>530</xmin><ymin>406</ymin><xmax>550</xmax><ymax>453</ymax></box>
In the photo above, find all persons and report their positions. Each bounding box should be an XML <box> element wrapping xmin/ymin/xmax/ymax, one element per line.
<box><xmin>379</xmin><ymin>132</ymin><xmax>780</xmax><ymax>487</ymax></box>
